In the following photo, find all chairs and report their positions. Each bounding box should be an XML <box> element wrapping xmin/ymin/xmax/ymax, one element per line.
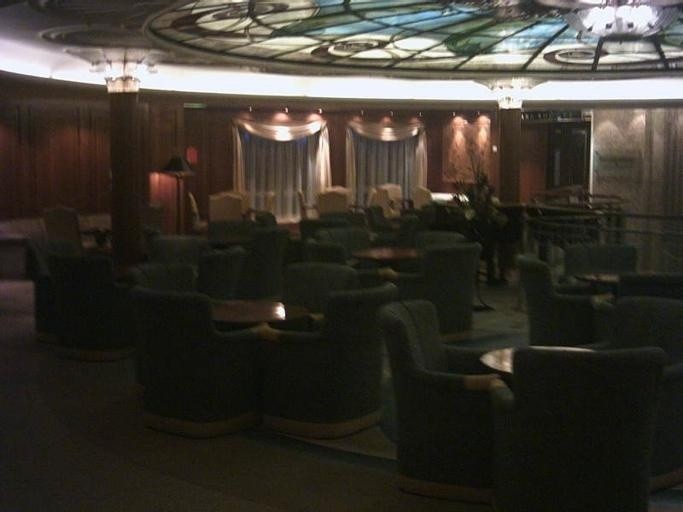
<box><xmin>22</xmin><ymin>184</ymin><xmax>683</xmax><ymax>512</ymax></box>
